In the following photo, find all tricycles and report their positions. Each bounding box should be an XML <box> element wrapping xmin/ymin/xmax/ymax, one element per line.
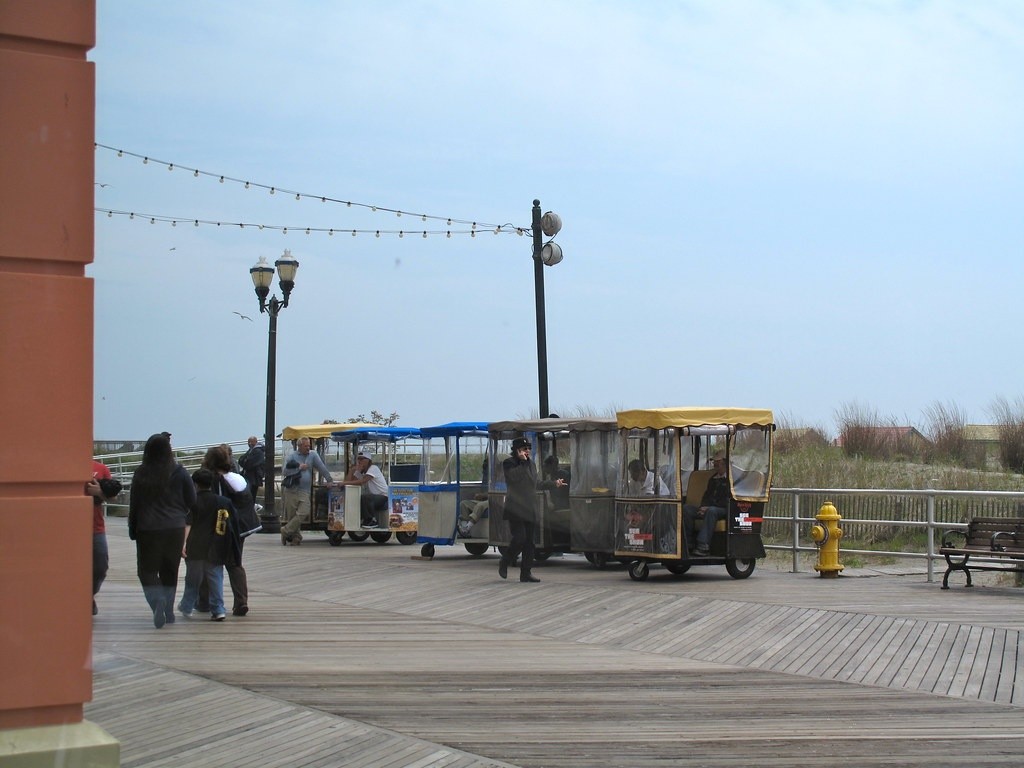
<box><xmin>612</xmin><ymin>408</ymin><xmax>776</xmax><ymax>581</ymax></box>
<box><xmin>279</xmin><ymin>421</ymin><xmax>425</xmax><ymax>546</ymax></box>
<box><xmin>484</xmin><ymin>419</ymin><xmax>624</xmax><ymax>568</ymax></box>
<box><xmin>417</xmin><ymin>421</ymin><xmax>538</xmax><ymax>558</ymax></box>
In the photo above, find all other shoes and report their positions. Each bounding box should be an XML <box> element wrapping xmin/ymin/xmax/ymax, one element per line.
<box><xmin>194</xmin><ymin>602</ymin><xmax>210</xmax><ymax>611</ymax></box>
<box><xmin>166</xmin><ymin>613</ymin><xmax>175</xmax><ymax>623</ymax></box>
<box><xmin>210</xmin><ymin>612</ymin><xmax>226</xmax><ymax>620</ymax></box>
<box><xmin>692</xmin><ymin>547</ymin><xmax>710</xmax><ymax>555</ymax></box>
<box><xmin>499</xmin><ymin>557</ymin><xmax>508</xmax><ymax>578</ymax></box>
<box><xmin>178</xmin><ymin>602</ymin><xmax>193</xmax><ymax>617</ymax></box>
<box><xmin>520</xmin><ymin>572</ymin><xmax>540</xmax><ymax>582</ymax></box>
<box><xmin>151</xmin><ymin>595</ymin><xmax>167</xmax><ymax>628</ymax></box>
<box><xmin>293</xmin><ymin>536</ymin><xmax>300</xmax><ymax>545</ymax></box>
<box><xmin>232</xmin><ymin>603</ymin><xmax>249</xmax><ymax>615</ymax></box>
<box><xmin>93</xmin><ymin>600</ymin><xmax>98</xmax><ymax>615</ymax></box>
<box><xmin>281</xmin><ymin>527</ymin><xmax>287</xmax><ymax>545</ymax></box>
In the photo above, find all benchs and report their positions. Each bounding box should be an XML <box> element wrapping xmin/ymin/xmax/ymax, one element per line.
<box><xmin>457</xmin><ymin>509</ymin><xmax>488</xmax><ymax>539</ymax></box>
<box><xmin>547</xmin><ymin>462</ymin><xmax>764</xmax><ymax>532</ymax></box>
<box><xmin>373</xmin><ymin>501</ymin><xmax>389</xmax><ymax>528</ymax></box>
<box><xmin>939</xmin><ymin>517</ymin><xmax>1024</xmax><ymax>592</ymax></box>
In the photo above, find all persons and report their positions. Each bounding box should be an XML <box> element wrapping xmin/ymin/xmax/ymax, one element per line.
<box><xmin>457</xmin><ymin>459</ymin><xmax>489</xmax><ymax>538</ymax></box>
<box><xmin>682</xmin><ymin>450</ymin><xmax>728</xmax><ymax>556</ymax></box>
<box><xmin>338</xmin><ymin>451</ymin><xmax>389</xmax><ymax>529</ymax></box>
<box><xmin>499</xmin><ymin>437</ymin><xmax>568</xmax><ymax>582</ymax></box>
<box><xmin>627</xmin><ymin>459</ymin><xmax>670</xmax><ymax>496</ymax></box>
<box><xmin>128</xmin><ymin>434</ymin><xmax>197</xmax><ymax>629</ymax></box>
<box><xmin>280</xmin><ymin>436</ymin><xmax>333</xmax><ymax>546</ymax></box>
<box><xmin>161</xmin><ymin>432</ymin><xmax>240</xmax><ymax>474</ymax></box>
<box><xmin>87</xmin><ymin>461</ymin><xmax>117</xmax><ymax>614</ymax></box>
<box><xmin>193</xmin><ymin>446</ymin><xmax>264</xmax><ymax>616</ymax></box>
<box><xmin>238</xmin><ymin>436</ymin><xmax>265</xmax><ymax>505</ymax></box>
<box><xmin>178</xmin><ymin>468</ymin><xmax>243</xmax><ymax>621</ymax></box>
<box><xmin>544</xmin><ymin>455</ymin><xmax>570</xmax><ymax>511</ymax></box>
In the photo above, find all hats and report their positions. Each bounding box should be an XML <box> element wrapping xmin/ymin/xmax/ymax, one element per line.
<box><xmin>511</xmin><ymin>438</ymin><xmax>531</xmax><ymax>449</ymax></box>
<box><xmin>358</xmin><ymin>451</ymin><xmax>372</xmax><ymax>460</ymax></box>
<box><xmin>710</xmin><ymin>449</ymin><xmax>726</xmax><ymax>461</ymax></box>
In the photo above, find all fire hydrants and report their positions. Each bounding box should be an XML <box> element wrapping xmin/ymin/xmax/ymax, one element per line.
<box><xmin>811</xmin><ymin>504</ymin><xmax>844</xmax><ymax>579</ymax></box>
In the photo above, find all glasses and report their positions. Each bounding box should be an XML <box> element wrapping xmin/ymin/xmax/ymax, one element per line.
<box><xmin>712</xmin><ymin>462</ymin><xmax>723</xmax><ymax>465</ymax></box>
<box><xmin>521</xmin><ymin>447</ymin><xmax>532</xmax><ymax>451</ymax></box>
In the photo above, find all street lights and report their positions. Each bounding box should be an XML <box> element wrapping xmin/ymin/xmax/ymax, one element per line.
<box><xmin>249</xmin><ymin>249</ymin><xmax>299</xmax><ymax>533</ymax></box>
<box><xmin>531</xmin><ymin>199</ymin><xmax>564</xmax><ymax>419</ymax></box>
